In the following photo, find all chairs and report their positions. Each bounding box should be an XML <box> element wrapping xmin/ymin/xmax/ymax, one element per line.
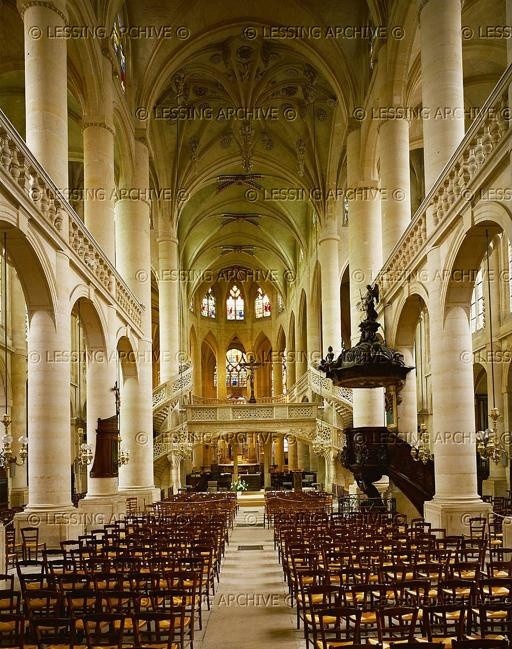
<box><xmin>263</xmin><ymin>489</ymin><xmax>511</xmax><ymax>648</ymax></box>
<box><xmin>0</xmin><ymin>486</ymin><xmax>238</xmax><ymax>648</ymax></box>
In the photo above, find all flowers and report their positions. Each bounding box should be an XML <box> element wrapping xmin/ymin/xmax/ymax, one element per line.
<box><xmin>231</xmin><ymin>479</ymin><xmax>249</xmax><ymax>492</ymax></box>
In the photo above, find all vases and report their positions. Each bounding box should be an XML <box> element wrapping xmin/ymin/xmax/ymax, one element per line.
<box><xmin>237</xmin><ymin>491</ymin><xmax>241</xmax><ymax>496</ymax></box>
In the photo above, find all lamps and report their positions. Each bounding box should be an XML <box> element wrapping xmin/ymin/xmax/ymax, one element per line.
<box><xmin>473</xmin><ymin>229</ymin><xmax>511</xmax><ymax>464</ymax></box>
<box><xmin>410</xmin><ymin>296</ymin><xmax>434</xmax><ymax>466</ymax></box>
<box><xmin>1</xmin><ymin>230</ymin><xmax>29</xmax><ymax>477</ymax></box>
